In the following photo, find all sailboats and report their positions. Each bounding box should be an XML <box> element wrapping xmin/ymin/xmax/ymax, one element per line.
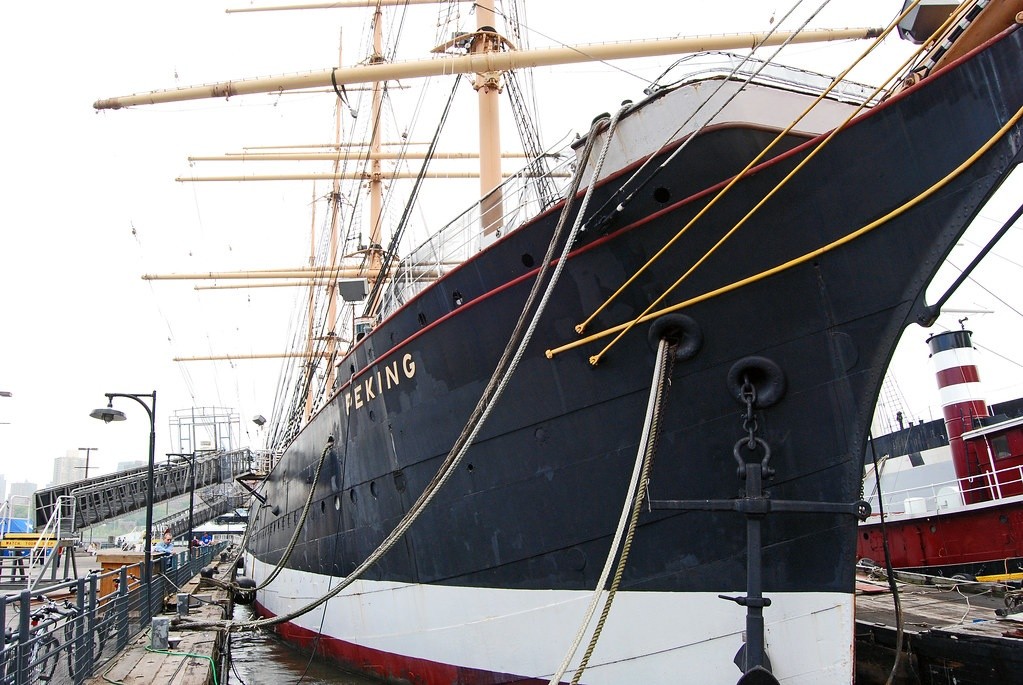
<box><xmin>92</xmin><ymin>0</ymin><xmax>1023</xmax><ymax>684</ymax></box>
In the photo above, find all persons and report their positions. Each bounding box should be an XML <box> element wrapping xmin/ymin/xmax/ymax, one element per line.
<box><xmin>190</xmin><ymin>530</ymin><xmax>214</xmax><ymax>548</ymax></box>
<box><xmin>57</xmin><ymin>546</ymin><xmax>63</xmax><ymax>566</ymax></box>
<box><xmin>153</xmin><ymin>531</ymin><xmax>178</xmax><ymax>572</ymax></box>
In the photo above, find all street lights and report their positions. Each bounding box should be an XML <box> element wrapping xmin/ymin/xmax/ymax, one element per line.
<box><xmin>89</xmin><ymin>391</ymin><xmax>156</xmax><ymax>623</ymax></box>
<box><xmin>159</xmin><ymin>451</ymin><xmax>195</xmax><ymax>561</ymax></box>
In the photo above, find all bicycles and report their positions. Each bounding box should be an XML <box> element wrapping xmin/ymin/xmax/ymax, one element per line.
<box><xmin>4</xmin><ymin>567</ymin><xmax>144</xmax><ymax>685</ymax></box>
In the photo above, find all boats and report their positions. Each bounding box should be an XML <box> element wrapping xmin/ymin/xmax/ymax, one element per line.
<box><xmin>857</xmin><ymin>319</ymin><xmax>1023</xmax><ymax>586</ymax></box>
